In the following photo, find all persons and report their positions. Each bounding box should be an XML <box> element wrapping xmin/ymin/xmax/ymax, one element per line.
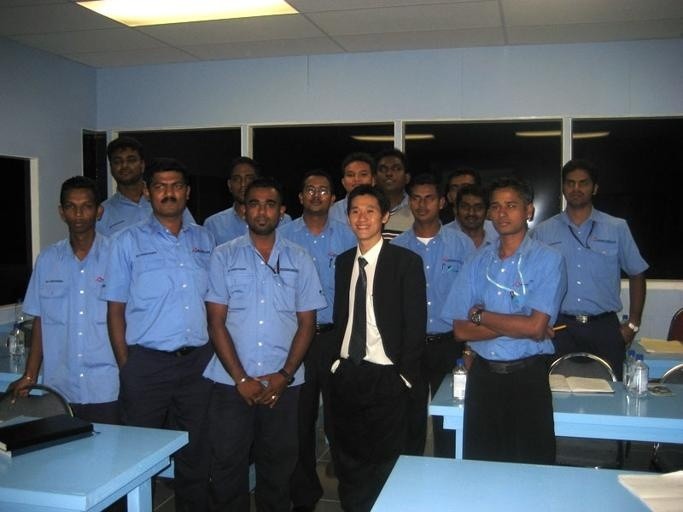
<box><xmin>5</xmin><ymin>176</ymin><xmax>119</xmax><ymax>424</ymax></box>
<box><xmin>440</xmin><ymin>179</ymin><xmax>567</xmax><ymax>465</ymax></box>
<box><xmin>97</xmin><ymin>164</ymin><xmax>215</xmax><ymax>512</ymax></box>
<box><xmin>326</xmin><ymin>184</ymin><xmax>427</xmax><ymax>512</ymax></box>
<box><xmin>95</xmin><ymin>136</ymin><xmax>197</xmax><ymax>241</ymax></box>
<box><xmin>531</xmin><ymin>158</ymin><xmax>650</xmax><ymax>382</ymax></box>
<box><xmin>448</xmin><ymin>171</ymin><xmax>480</xmax><ymax>222</ymax></box>
<box><xmin>386</xmin><ymin>176</ymin><xmax>476</xmax><ymax>457</ymax></box>
<box><xmin>375</xmin><ymin>149</ymin><xmax>415</xmax><ymax>242</ymax></box>
<box><xmin>200</xmin><ymin>180</ymin><xmax>327</xmax><ymax>511</ymax></box>
<box><xmin>279</xmin><ymin>171</ymin><xmax>357</xmax><ymax>511</ymax></box>
<box><xmin>326</xmin><ymin>156</ymin><xmax>375</xmax><ymax>227</ymax></box>
<box><xmin>443</xmin><ymin>185</ymin><xmax>499</xmax><ymax>251</ymax></box>
<box><xmin>204</xmin><ymin>156</ymin><xmax>292</xmax><ymax>248</ymax></box>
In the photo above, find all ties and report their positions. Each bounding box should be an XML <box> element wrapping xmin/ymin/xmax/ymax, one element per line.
<box><xmin>348</xmin><ymin>256</ymin><xmax>368</xmax><ymax>366</ymax></box>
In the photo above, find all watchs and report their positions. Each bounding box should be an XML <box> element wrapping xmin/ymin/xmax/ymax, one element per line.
<box><xmin>470</xmin><ymin>309</ymin><xmax>484</xmax><ymax>326</ymax></box>
<box><xmin>278</xmin><ymin>367</ymin><xmax>295</xmax><ymax>386</ymax></box>
<box><xmin>625</xmin><ymin>322</ymin><xmax>639</xmax><ymax>333</ymax></box>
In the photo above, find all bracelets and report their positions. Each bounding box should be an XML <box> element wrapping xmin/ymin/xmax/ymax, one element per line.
<box><xmin>23</xmin><ymin>375</ymin><xmax>38</xmax><ymax>383</ymax></box>
<box><xmin>234</xmin><ymin>375</ymin><xmax>249</xmax><ymax>387</ymax></box>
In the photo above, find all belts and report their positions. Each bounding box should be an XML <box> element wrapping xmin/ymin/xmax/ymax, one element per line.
<box><xmin>172</xmin><ymin>345</ymin><xmax>196</xmax><ymax>357</ymax></box>
<box><xmin>315</xmin><ymin>322</ymin><xmax>334</xmax><ymax>335</ymax></box>
<box><xmin>423</xmin><ymin>331</ymin><xmax>454</xmax><ymax>345</ymax></box>
<box><xmin>472</xmin><ymin>355</ymin><xmax>540</xmax><ymax>372</ymax></box>
<box><xmin>559</xmin><ymin>310</ymin><xmax>616</xmax><ymax>324</ymax></box>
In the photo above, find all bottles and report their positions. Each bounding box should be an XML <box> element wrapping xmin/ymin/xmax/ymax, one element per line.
<box><xmin>622</xmin><ymin>314</ymin><xmax>628</xmax><ymax>325</ymax></box>
<box><xmin>453</xmin><ymin>357</ymin><xmax>468</xmax><ymax>401</ymax></box>
<box><xmin>630</xmin><ymin>354</ymin><xmax>649</xmax><ymax>399</ymax></box>
<box><xmin>623</xmin><ymin>350</ymin><xmax>636</xmax><ymax>387</ymax></box>
<box><xmin>7</xmin><ymin>321</ymin><xmax>25</xmax><ymax>356</ymax></box>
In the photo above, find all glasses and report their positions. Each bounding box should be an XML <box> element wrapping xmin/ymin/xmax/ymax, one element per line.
<box><xmin>380</xmin><ymin>165</ymin><xmax>401</xmax><ymax>173</ymax></box>
<box><xmin>307</xmin><ymin>187</ymin><xmax>329</xmax><ymax>197</ymax></box>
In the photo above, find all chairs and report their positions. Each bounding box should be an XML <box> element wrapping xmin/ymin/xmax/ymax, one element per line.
<box><xmin>5</xmin><ymin>383</ymin><xmax>75</xmax><ymax>419</ymax></box>
<box><xmin>547</xmin><ymin>353</ymin><xmax>625</xmax><ymax>465</ymax></box>
<box><xmin>651</xmin><ymin>363</ymin><xmax>683</xmax><ymax>472</ymax></box>
<box><xmin>667</xmin><ymin>309</ymin><xmax>683</xmax><ymax>340</ymax></box>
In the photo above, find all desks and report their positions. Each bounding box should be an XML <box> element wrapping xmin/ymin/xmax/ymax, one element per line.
<box><xmin>372</xmin><ymin>453</ymin><xmax>681</xmax><ymax>507</ymax></box>
<box><xmin>2</xmin><ymin>318</ymin><xmax>44</xmax><ymax>396</ymax></box>
<box><xmin>629</xmin><ymin>338</ymin><xmax>683</xmax><ymax>382</ymax></box>
<box><xmin>427</xmin><ymin>369</ymin><xmax>682</xmax><ymax>461</ymax></box>
<box><xmin>2</xmin><ymin>415</ymin><xmax>189</xmax><ymax>507</ymax></box>
